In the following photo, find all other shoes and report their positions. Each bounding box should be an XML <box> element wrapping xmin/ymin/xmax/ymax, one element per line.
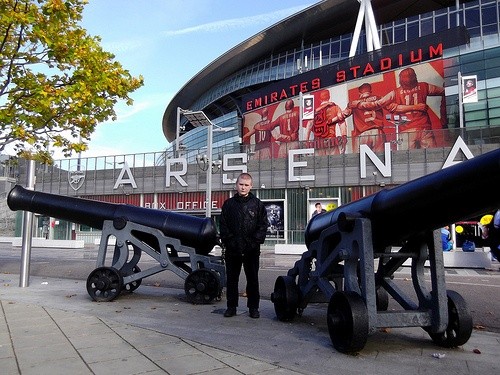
<box><xmin>249</xmin><ymin>308</ymin><xmax>259</xmax><ymax>318</ymax></box>
<box><xmin>224</xmin><ymin>306</ymin><xmax>236</xmax><ymax>317</ymax></box>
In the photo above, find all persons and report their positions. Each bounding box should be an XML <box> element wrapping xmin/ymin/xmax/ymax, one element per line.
<box><xmin>312</xmin><ymin>203</ymin><xmax>327</xmax><ymax>218</ymax></box>
<box><xmin>346</xmin><ymin>68</ymin><xmax>446</xmax><ymax>152</ymax></box>
<box><xmin>440</xmin><ymin>224</ymin><xmax>454</xmax><ymax>251</ymax></box>
<box><xmin>218</xmin><ymin>173</ymin><xmax>267</xmax><ymax>318</ymax></box>
<box><xmin>252</xmin><ymin>100</ymin><xmax>335</xmax><ymax>158</ymax></box>
<box><xmin>306</xmin><ymin>90</ymin><xmax>348</xmax><ymax>158</ymax></box>
<box><xmin>241</xmin><ymin>107</ymin><xmax>291</xmax><ymax>162</ymax></box>
<box><xmin>325</xmin><ymin>84</ymin><xmax>430</xmax><ymax>154</ymax></box>
<box><xmin>462</xmin><ymin>79</ymin><xmax>476</xmax><ymax>96</ymax></box>
